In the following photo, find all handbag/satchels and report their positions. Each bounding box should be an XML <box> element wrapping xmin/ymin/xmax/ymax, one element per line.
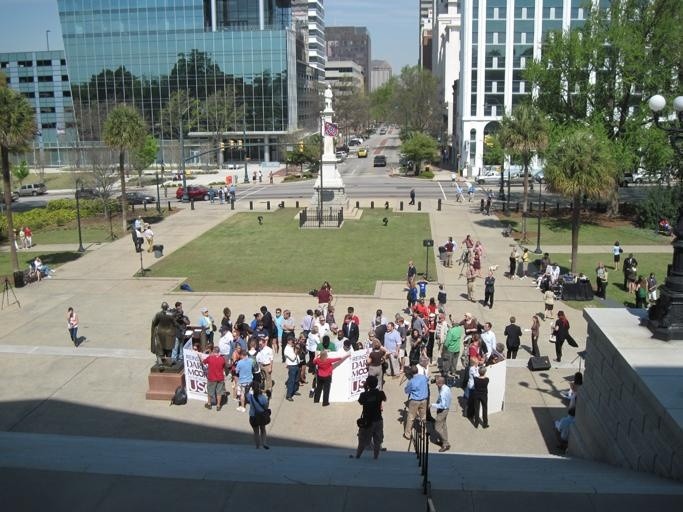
<box><xmin>173</xmin><ymin>387</ymin><xmax>186</xmax><ymax>404</ymax></box>
<box><xmin>249</xmin><ymin>395</ymin><xmax>270</xmax><ymax>426</ymax></box>
<box><xmin>356</xmin><ymin>412</ymin><xmax>372</xmax><ymax>428</ymax></box>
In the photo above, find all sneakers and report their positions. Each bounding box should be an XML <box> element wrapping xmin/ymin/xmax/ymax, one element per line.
<box><xmin>216</xmin><ymin>406</ymin><xmax>222</xmax><ymax>411</ymax></box>
<box><xmin>204</xmin><ymin>403</ymin><xmax>211</xmax><ymax>409</ymax></box>
<box><xmin>285</xmin><ymin>376</ymin><xmax>305</xmax><ymax>401</ymax></box>
<box><xmin>236</xmin><ymin>407</ymin><xmax>245</xmax><ymax>412</ymax></box>
<box><xmin>314</xmin><ymin>399</ymin><xmax>329</xmax><ymax>406</ymax></box>
<box><xmin>256</xmin><ymin>444</ymin><xmax>269</xmax><ymax>450</ymax></box>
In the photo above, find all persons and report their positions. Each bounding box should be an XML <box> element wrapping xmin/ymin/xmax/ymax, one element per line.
<box><xmin>409</xmin><ymin>188</ymin><xmax>415</xmax><ymax>205</ymax></box>
<box><xmin>443</xmin><ymin>234</ymin><xmax>658</xmax><ymax>309</ymax></box>
<box><xmin>67</xmin><ymin>306</ymin><xmax>78</xmax><ymax>346</ymax></box>
<box><xmin>660</xmin><ymin>218</ymin><xmax>672</xmax><ymax>233</ymax></box>
<box><xmin>209</xmin><ymin>170</ymin><xmax>262</xmax><ymax>202</ymax></box>
<box><xmin>172</xmin><ymin>282</ymin><xmax>569</xmax><ymax>458</ymax></box>
<box><xmin>152</xmin><ymin>303</ymin><xmax>176</xmax><ymax>368</ymax></box>
<box><xmin>132</xmin><ymin>216</ymin><xmax>154</xmax><ymax>252</ymax></box>
<box><xmin>13</xmin><ymin>226</ymin><xmax>56</xmax><ymax>283</ymax></box>
<box><xmin>452</xmin><ymin>173</ymin><xmax>494</xmax><ymax>215</ymax></box>
<box><xmin>173</xmin><ymin>171</ymin><xmax>184</xmax><ymax>180</ymax></box>
<box><xmin>407</xmin><ymin>261</ymin><xmax>417</xmax><ymax>289</ymax></box>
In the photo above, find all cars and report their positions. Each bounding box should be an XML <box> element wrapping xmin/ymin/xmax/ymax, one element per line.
<box><xmin>373</xmin><ymin>155</ymin><xmax>387</xmax><ymax>167</ymax></box>
<box><xmin>335</xmin><ymin>120</ymin><xmax>378</xmax><ymax>163</ymax></box>
<box><xmin>380</xmin><ymin>123</ymin><xmax>400</xmax><ymax>135</ymax></box>
<box><xmin>0</xmin><ymin>192</ymin><xmax>19</xmax><ymax>202</ymax></box>
<box><xmin>399</xmin><ymin>151</ymin><xmax>431</xmax><ymax>168</ymax></box>
<box><xmin>536</xmin><ymin>169</ymin><xmax>549</xmax><ymax>184</ymax></box>
<box><xmin>117</xmin><ymin>192</ymin><xmax>155</xmax><ymax>205</ymax></box>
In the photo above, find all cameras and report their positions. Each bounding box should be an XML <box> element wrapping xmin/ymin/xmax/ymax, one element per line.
<box><xmin>309</xmin><ymin>288</ymin><xmax>318</xmax><ymax>297</ymax></box>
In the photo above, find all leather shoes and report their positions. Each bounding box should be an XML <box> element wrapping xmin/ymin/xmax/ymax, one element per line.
<box><xmin>439</xmin><ymin>446</ymin><xmax>450</xmax><ymax>452</ymax></box>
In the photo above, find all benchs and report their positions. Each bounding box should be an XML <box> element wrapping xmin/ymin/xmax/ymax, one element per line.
<box><xmin>21</xmin><ymin>268</ymin><xmax>44</xmax><ymax>282</ymax></box>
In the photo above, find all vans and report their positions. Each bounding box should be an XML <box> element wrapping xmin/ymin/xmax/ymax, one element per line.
<box><xmin>75</xmin><ymin>187</ymin><xmax>104</xmax><ymax>199</ymax></box>
<box><xmin>13</xmin><ymin>183</ymin><xmax>47</xmax><ymax>198</ymax></box>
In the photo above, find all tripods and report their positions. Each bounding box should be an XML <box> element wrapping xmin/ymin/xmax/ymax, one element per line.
<box><xmin>1</xmin><ymin>277</ymin><xmax>20</xmax><ymax>309</ymax></box>
<box><xmin>137</xmin><ymin>252</ymin><xmax>150</xmax><ymax>277</ymax></box>
<box><xmin>458</xmin><ymin>252</ymin><xmax>468</xmax><ymax>277</ymax></box>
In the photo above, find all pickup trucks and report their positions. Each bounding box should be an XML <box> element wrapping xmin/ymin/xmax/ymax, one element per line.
<box><xmin>474</xmin><ymin>171</ymin><xmax>508</xmax><ymax>185</ymax></box>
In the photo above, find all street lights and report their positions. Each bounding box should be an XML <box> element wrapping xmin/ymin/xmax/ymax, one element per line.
<box><xmin>645</xmin><ymin>90</ymin><xmax>682</xmax><ymax>338</ymax></box>
<box><xmin>422</xmin><ymin>237</ymin><xmax>436</xmax><ymax>282</ymax></box>
<box><xmin>134</xmin><ymin>235</ymin><xmax>148</xmax><ymax>278</ymax></box>
<box><xmin>652</xmin><ymin>174</ymin><xmax>662</xmax><ymax>234</ymax></box>
<box><xmin>73</xmin><ymin>178</ymin><xmax>87</xmax><ymax>253</ymax></box>
<box><xmin>395</xmin><ymin>103</ymin><xmax>411</xmax><ymax>142</ymax></box>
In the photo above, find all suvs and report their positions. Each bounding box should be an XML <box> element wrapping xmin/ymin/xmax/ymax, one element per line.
<box><xmin>612</xmin><ymin>168</ymin><xmax>668</xmax><ymax>188</ymax></box>
<box><xmin>176</xmin><ymin>185</ymin><xmax>218</xmax><ymax>202</ymax></box>
<box><xmin>499</xmin><ymin>172</ymin><xmax>534</xmax><ymax>186</ymax></box>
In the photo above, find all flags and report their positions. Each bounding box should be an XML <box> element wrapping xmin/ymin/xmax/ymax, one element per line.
<box><xmin>321</xmin><ymin>118</ymin><xmax>339</xmax><ymax>137</ymax></box>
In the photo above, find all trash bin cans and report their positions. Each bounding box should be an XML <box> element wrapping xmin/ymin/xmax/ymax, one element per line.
<box><xmin>153</xmin><ymin>245</ymin><xmax>163</xmax><ymax>258</ymax></box>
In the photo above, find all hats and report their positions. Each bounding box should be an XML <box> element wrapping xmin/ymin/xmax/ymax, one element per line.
<box><xmin>200</xmin><ymin>307</ymin><xmax>209</xmax><ymax>314</ymax></box>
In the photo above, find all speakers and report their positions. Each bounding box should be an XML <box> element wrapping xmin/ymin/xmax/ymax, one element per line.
<box><xmin>135</xmin><ymin>237</ymin><xmax>144</xmax><ymax>252</ymax></box>
<box><xmin>528</xmin><ymin>356</ymin><xmax>551</xmax><ymax>371</ymax></box>
<box><xmin>423</xmin><ymin>240</ymin><xmax>433</xmax><ymax>246</ymax></box>
